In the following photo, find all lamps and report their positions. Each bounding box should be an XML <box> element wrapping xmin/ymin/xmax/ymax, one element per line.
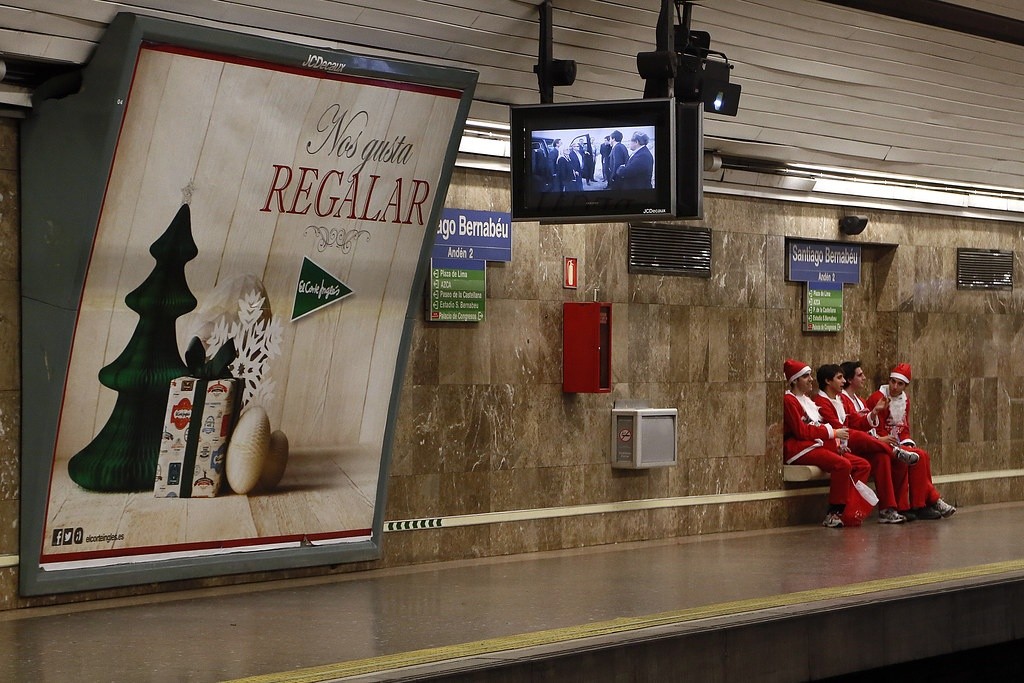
<box><xmin>635</xmin><ymin>27</ymin><xmax>743</xmax><ymax>120</ymax></box>
<box><xmin>839</xmin><ymin>215</ymin><xmax>868</xmax><ymax>237</ymax></box>
<box><xmin>548</xmin><ymin>58</ymin><xmax>579</xmax><ymax>86</ymax></box>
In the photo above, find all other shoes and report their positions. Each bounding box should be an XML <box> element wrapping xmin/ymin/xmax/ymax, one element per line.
<box><xmin>591</xmin><ymin>179</ymin><xmax>598</xmax><ymax>182</ymax></box>
<box><xmin>603</xmin><ymin>186</ymin><xmax>609</xmax><ymax>189</ymax></box>
<box><xmin>602</xmin><ymin>179</ymin><xmax>606</xmax><ymax>182</ymax></box>
<box><xmin>587</xmin><ymin>182</ymin><xmax>590</xmax><ymax>185</ymax></box>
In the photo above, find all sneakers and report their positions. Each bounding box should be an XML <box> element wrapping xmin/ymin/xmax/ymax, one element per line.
<box><xmin>898</xmin><ymin>511</ymin><xmax>916</xmax><ymax>522</ymax></box>
<box><xmin>890</xmin><ymin>444</ymin><xmax>919</xmax><ymax>465</ymax></box>
<box><xmin>878</xmin><ymin>510</ymin><xmax>907</xmax><ymax>523</ymax></box>
<box><xmin>822</xmin><ymin>514</ymin><xmax>844</xmax><ymax>528</ymax></box>
<box><xmin>909</xmin><ymin>508</ymin><xmax>936</xmax><ymax>519</ymax></box>
<box><xmin>933</xmin><ymin>499</ymin><xmax>957</xmax><ymax>517</ymax></box>
<box><xmin>928</xmin><ymin>506</ymin><xmax>941</xmax><ymax>518</ymax></box>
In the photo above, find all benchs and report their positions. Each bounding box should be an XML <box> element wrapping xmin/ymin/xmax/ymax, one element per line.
<box><xmin>782</xmin><ymin>464</ymin><xmax>832</xmax><ymax>483</ymax></box>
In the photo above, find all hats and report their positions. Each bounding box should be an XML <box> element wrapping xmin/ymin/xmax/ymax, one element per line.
<box><xmin>890</xmin><ymin>363</ymin><xmax>912</xmax><ymax>384</ymax></box>
<box><xmin>783</xmin><ymin>359</ymin><xmax>812</xmax><ymax>385</ymax></box>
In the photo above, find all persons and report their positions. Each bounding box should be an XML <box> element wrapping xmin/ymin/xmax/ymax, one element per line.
<box><xmin>533</xmin><ymin>130</ymin><xmax>653</xmax><ymax>191</ymax></box>
<box><xmin>783</xmin><ymin>359</ymin><xmax>957</xmax><ymax>527</ymax></box>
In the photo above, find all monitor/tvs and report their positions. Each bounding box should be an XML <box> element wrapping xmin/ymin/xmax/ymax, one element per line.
<box><xmin>509</xmin><ymin>97</ymin><xmax>704</xmax><ymax>226</ymax></box>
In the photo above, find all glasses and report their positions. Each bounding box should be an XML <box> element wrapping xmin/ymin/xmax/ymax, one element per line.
<box><xmin>574</xmin><ymin>146</ymin><xmax>579</xmax><ymax>147</ymax></box>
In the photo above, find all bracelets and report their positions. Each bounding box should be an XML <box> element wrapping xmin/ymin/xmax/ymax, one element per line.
<box><xmin>873</xmin><ymin>407</ymin><xmax>879</xmax><ymax>414</ymax></box>
<box><xmin>833</xmin><ymin>428</ymin><xmax>837</xmax><ymax>438</ymax></box>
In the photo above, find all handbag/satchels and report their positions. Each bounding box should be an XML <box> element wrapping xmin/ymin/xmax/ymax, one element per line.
<box><xmin>839</xmin><ymin>475</ymin><xmax>880</xmax><ymax>527</ymax></box>
